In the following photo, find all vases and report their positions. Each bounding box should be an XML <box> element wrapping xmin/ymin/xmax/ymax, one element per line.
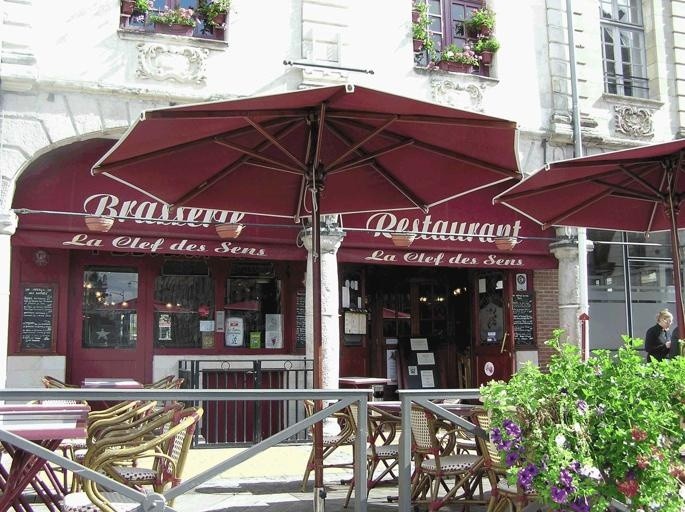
<box><xmin>121</xmin><ymin>0</ymin><xmax>135</xmax><ymax>15</ymax></box>
<box><xmin>155</xmin><ymin>22</ymin><xmax>194</xmax><ymax>36</ymax></box>
<box><xmin>437</xmin><ymin>60</ymin><xmax>473</xmax><ymax>73</ymax></box>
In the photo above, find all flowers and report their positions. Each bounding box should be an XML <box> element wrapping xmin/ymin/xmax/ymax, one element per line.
<box><xmin>132</xmin><ymin>0</ymin><xmax>229</xmax><ymax>30</ymax></box>
<box><xmin>412</xmin><ymin>0</ymin><xmax>499</xmax><ymax>63</ymax></box>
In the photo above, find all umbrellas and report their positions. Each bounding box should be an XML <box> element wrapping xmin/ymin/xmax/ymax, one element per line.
<box><xmin>491</xmin><ymin>137</ymin><xmax>685</xmax><ymax>343</ymax></box>
<box><xmin>91</xmin><ymin>82</ymin><xmax>521</xmax><ymax>487</ymax></box>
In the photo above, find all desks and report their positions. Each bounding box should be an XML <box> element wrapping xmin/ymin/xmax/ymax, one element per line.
<box><xmin>340</xmin><ymin>376</ymin><xmax>391</xmax><ymax>403</ymax></box>
<box><xmin>0</xmin><ymin>400</ymin><xmax>89</xmax><ymax>512</ymax></box>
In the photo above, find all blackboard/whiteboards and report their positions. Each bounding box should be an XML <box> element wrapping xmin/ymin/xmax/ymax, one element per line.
<box><xmin>292</xmin><ymin>289</ymin><xmax>306</xmax><ymax>355</ymax></box>
<box><xmin>17</xmin><ymin>283</ymin><xmax>60</xmax><ymax>353</ymax></box>
<box><xmin>511</xmin><ymin>292</ymin><xmax>539</xmax><ymax>347</ymax></box>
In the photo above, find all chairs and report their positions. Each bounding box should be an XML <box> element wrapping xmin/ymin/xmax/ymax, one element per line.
<box><xmin>303</xmin><ymin>399</ymin><xmax>544</xmax><ymax>512</ymax></box>
<box><xmin>42</xmin><ymin>374</ymin><xmax>204</xmax><ymax>511</ymax></box>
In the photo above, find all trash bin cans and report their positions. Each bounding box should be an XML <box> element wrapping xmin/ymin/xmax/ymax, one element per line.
<box><xmin>369</xmin><ymin>385</ymin><xmax>386</xmax><ymax>401</ymax></box>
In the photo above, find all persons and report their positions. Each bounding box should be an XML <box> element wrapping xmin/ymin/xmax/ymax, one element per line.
<box><xmin>646</xmin><ymin>309</ymin><xmax>675</xmax><ymax>359</ymax></box>
